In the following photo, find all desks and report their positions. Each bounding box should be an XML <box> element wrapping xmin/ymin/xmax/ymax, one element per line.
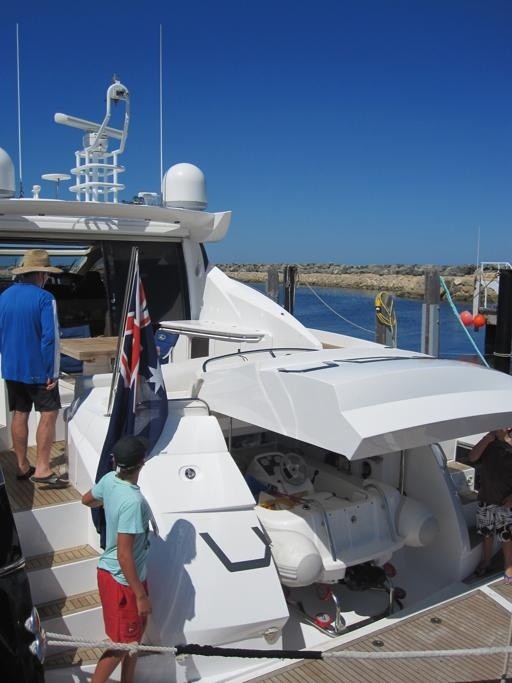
<box><xmin>60</xmin><ymin>336</ymin><xmax>120</xmax><ymax>375</ymax></box>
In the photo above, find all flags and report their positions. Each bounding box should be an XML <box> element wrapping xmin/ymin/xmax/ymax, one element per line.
<box><xmin>90</xmin><ymin>246</ymin><xmax>169</xmax><ymax>551</ymax></box>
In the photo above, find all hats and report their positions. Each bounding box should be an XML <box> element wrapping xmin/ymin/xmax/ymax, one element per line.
<box><xmin>10</xmin><ymin>249</ymin><xmax>63</xmax><ymax>274</ymax></box>
<box><xmin>113</xmin><ymin>435</ymin><xmax>149</xmax><ymax>468</ymax></box>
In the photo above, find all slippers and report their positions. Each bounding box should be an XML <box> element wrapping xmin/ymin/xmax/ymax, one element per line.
<box><xmin>504</xmin><ymin>572</ymin><xmax>512</xmax><ymax>583</ymax></box>
<box><xmin>477</xmin><ymin>562</ymin><xmax>491</xmax><ymax>577</ymax></box>
<box><xmin>16</xmin><ymin>467</ymin><xmax>70</xmax><ymax>485</ymax></box>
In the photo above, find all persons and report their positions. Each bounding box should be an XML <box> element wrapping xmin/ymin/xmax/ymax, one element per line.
<box><xmin>0</xmin><ymin>249</ymin><xmax>69</xmax><ymax>484</ymax></box>
<box><xmin>469</xmin><ymin>424</ymin><xmax>512</xmax><ymax>584</ymax></box>
<box><xmin>82</xmin><ymin>435</ymin><xmax>152</xmax><ymax>683</ymax></box>
